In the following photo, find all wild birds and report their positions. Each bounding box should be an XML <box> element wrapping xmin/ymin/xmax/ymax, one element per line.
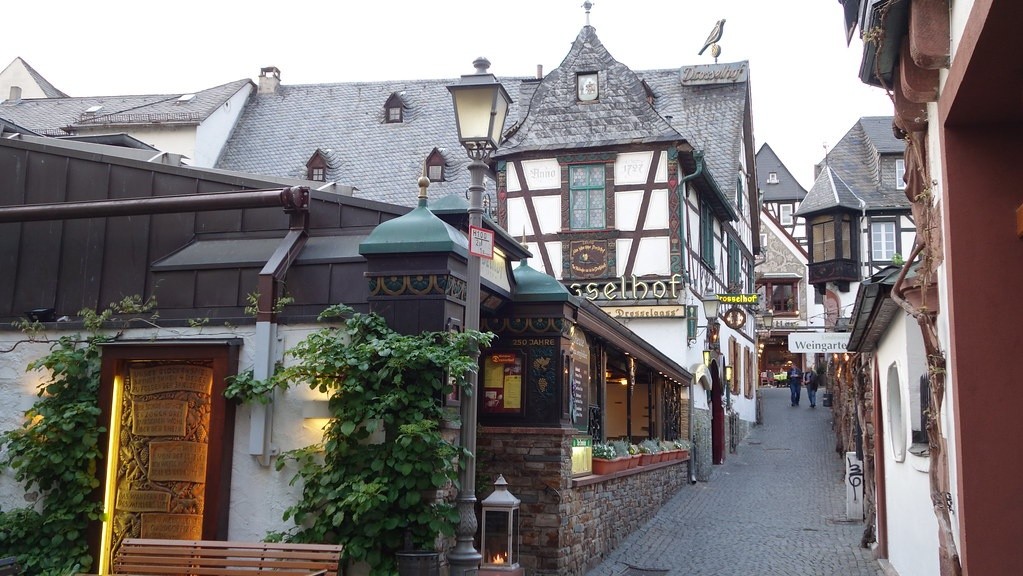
<box><xmin>698</xmin><ymin>19</ymin><xmax>726</xmax><ymax>55</ymax></box>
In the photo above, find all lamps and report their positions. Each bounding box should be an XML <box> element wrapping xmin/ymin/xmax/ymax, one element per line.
<box><xmin>755</xmin><ymin>309</ymin><xmax>774</xmax><ymax>336</ymax></box>
<box><xmin>686</xmin><ymin>286</ymin><xmax>721</xmax><ymax>348</ymax></box>
<box><xmin>24</xmin><ymin>308</ymin><xmax>57</xmax><ymax>322</ymax></box>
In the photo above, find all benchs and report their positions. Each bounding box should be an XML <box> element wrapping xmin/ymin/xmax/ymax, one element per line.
<box><xmin>110</xmin><ymin>538</ymin><xmax>343</xmax><ymax>576</ymax></box>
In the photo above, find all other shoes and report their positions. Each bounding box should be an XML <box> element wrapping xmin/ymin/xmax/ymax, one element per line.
<box><xmin>791</xmin><ymin>402</ymin><xmax>799</xmax><ymax>407</ymax></box>
<box><xmin>810</xmin><ymin>403</ymin><xmax>816</xmax><ymax>408</ymax></box>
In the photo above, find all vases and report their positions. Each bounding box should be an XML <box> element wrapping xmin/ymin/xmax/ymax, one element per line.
<box><xmin>395</xmin><ymin>549</ymin><xmax>440</xmax><ymax>576</ymax></box>
<box><xmin>592</xmin><ymin>450</ymin><xmax>689</xmax><ymax>475</ymax></box>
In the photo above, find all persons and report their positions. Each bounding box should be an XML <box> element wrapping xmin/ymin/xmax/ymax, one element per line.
<box><xmin>804</xmin><ymin>367</ymin><xmax>818</xmax><ymax>407</ymax></box>
<box><xmin>787</xmin><ymin>362</ymin><xmax>804</xmax><ymax>407</ymax></box>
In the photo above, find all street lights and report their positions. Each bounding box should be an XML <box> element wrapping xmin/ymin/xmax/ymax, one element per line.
<box><xmin>445</xmin><ymin>55</ymin><xmax>513</xmax><ymax>576</ymax></box>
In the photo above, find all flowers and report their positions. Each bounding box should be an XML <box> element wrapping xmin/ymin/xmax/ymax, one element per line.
<box><xmin>592</xmin><ymin>437</ymin><xmax>694</xmax><ymax>460</ymax></box>
